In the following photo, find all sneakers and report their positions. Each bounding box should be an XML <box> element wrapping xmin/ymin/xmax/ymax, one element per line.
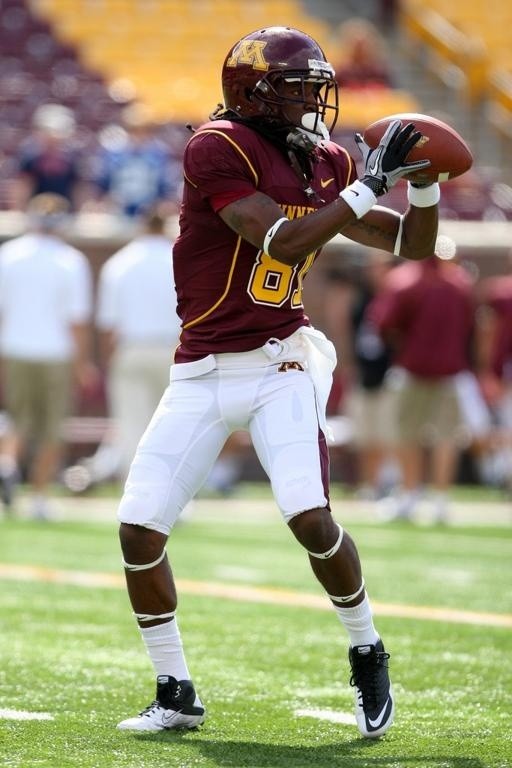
<box><xmin>116</xmin><ymin>674</ymin><xmax>205</xmax><ymax>732</ymax></box>
<box><xmin>348</xmin><ymin>637</ymin><xmax>394</xmax><ymax>738</ymax></box>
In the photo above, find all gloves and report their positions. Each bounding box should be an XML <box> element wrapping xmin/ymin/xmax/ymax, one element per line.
<box><xmin>355</xmin><ymin>120</ymin><xmax>431</xmax><ymax>198</ymax></box>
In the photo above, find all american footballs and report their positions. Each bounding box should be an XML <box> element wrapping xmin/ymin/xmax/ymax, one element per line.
<box><xmin>363</xmin><ymin>113</ymin><xmax>473</xmax><ymax>183</ymax></box>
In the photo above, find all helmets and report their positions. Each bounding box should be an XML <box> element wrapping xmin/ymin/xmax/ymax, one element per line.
<box><xmin>221</xmin><ymin>27</ymin><xmax>335</xmax><ymax>117</ymax></box>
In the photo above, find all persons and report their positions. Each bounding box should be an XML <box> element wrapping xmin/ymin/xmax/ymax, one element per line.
<box><xmin>113</xmin><ymin>27</ymin><xmax>444</xmax><ymax>740</ymax></box>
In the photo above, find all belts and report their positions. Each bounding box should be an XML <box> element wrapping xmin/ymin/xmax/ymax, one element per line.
<box><xmin>169</xmin><ymin>328</ymin><xmax>304</xmax><ymax>381</ymax></box>
